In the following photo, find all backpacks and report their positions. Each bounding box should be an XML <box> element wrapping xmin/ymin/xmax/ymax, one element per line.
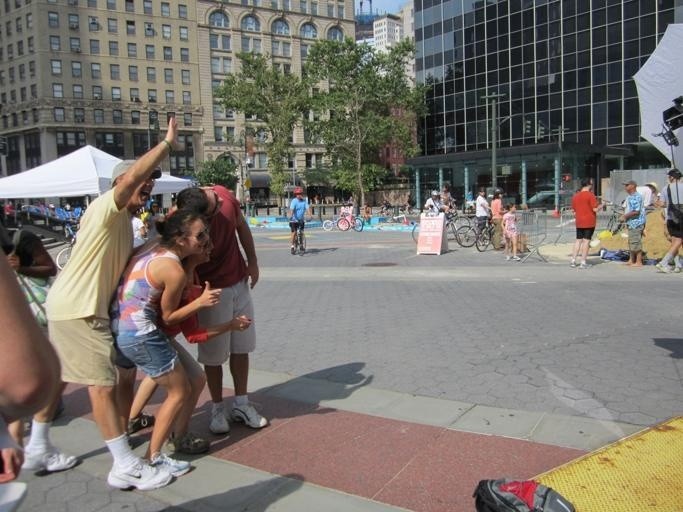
<box><xmin>473</xmin><ymin>477</ymin><xmax>575</xmax><ymax>512</ymax></box>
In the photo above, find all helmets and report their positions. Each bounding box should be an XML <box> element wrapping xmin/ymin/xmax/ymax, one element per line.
<box><xmin>294</xmin><ymin>188</ymin><xmax>303</xmax><ymax>195</ymax></box>
<box><xmin>494</xmin><ymin>188</ymin><xmax>502</xmax><ymax>194</ymax></box>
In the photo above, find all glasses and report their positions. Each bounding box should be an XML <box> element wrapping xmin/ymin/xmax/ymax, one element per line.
<box><xmin>205</xmin><ymin>192</ymin><xmax>218</xmax><ymax>219</ymax></box>
<box><xmin>183</xmin><ymin>227</ymin><xmax>209</xmax><ymax>240</ymax></box>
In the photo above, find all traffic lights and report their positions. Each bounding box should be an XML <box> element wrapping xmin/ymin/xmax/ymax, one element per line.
<box><xmin>537</xmin><ymin>124</ymin><xmax>544</xmax><ymax>140</ymax></box>
<box><xmin>523</xmin><ymin>120</ymin><xmax>531</xmax><ymax>134</ymax></box>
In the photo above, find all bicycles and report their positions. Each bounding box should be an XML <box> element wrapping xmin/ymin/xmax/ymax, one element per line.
<box><xmin>291</xmin><ymin>222</ymin><xmax>307</xmax><ymax>256</ymax></box>
<box><xmin>55</xmin><ymin>245</ymin><xmax>73</xmax><ymax>269</ymax></box>
<box><xmin>606</xmin><ymin>207</ymin><xmax>623</xmax><ymax>234</ymax></box>
<box><xmin>320</xmin><ymin>209</ymin><xmax>364</xmax><ymax>231</ymax></box>
<box><xmin>408</xmin><ymin>197</ymin><xmax>498</xmax><ymax>251</ymax></box>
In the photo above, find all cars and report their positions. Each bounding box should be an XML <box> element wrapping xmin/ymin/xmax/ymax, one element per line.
<box><xmin>465</xmin><ymin>187</ymin><xmax>515</xmax><ymax>210</ymax></box>
<box><xmin>525</xmin><ymin>190</ymin><xmax>575</xmax><ymax>211</ymax></box>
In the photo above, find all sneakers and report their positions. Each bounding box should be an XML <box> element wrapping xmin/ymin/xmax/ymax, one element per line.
<box><xmin>505</xmin><ymin>256</ymin><xmax>510</xmax><ymax>261</ymax></box>
<box><xmin>579</xmin><ymin>263</ymin><xmax>591</xmax><ymax>269</ymax></box>
<box><xmin>655</xmin><ymin>262</ymin><xmax>666</xmax><ymax>273</ymax></box>
<box><xmin>122</xmin><ymin>412</ymin><xmax>151</xmax><ymax>435</ymax></box>
<box><xmin>290</xmin><ymin>248</ymin><xmax>295</xmax><ymax>254</ymax></box>
<box><xmin>512</xmin><ymin>256</ymin><xmax>519</xmax><ymax>260</ymax></box>
<box><xmin>20</xmin><ymin>449</ymin><xmax>78</xmax><ymax>471</ymax></box>
<box><xmin>108</xmin><ymin>432</ymin><xmax>209</xmax><ymax>490</ymax></box>
<box><xmin>230</xmin><ymin>400</ymin><xmax>267</xmax><ymax>428</ymax></box>
<box><xmin>570</xmin><ymin>261</ymin><xmax>575</xmax><ymax>267</ymax></box>
<box><xmin>209</xmin><ymin>409</ymin><xmax>230</xmax><ymax>435</ymax></box>
<box><xmin>674</xmin><ymin>267</ymin><xmax>680</xmax><ymax>272</ymax></box>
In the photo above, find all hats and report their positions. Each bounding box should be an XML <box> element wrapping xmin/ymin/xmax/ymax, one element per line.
<box><xmin>431</xmin><ymin>191</ymin><xmax>439</xmax><ymax>196</ymax></box>
<box><xmin>646</xmin><ymin>183</ymin><xmax>658</xmax><ymax>193</ymax></box>
<box><xmin>109</xmin><ymin>159</ymin><xmax>161</xmax><ymax>187</ymax></box>
<box><xmin>622</xmin><ymin>180</ymin><xmax>636</xmax><ymax>186</ymax></box>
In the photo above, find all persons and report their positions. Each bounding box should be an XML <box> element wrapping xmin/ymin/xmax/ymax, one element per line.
<box><xmin>500</xmin><ymin>203</ymin><xmax>523</xmax><ymax>261</ymax></box>
<box><xmin>1</xmin><ymin>118</ymin><xmax>267</xmax><ymax>511</ymax></box>
<box><xmin>403</xmin><ymin>195</ymin><xmax>414</xmax><ymax>215</ymax></box>
<box><xmin>363</xmin><ymin>202</ymin><xmax>373</xmax><ymax>218</ymax></box>
<box><xmin>440</xmin><ymin>184</ymin><xmax>456</xmax><ymax>207</ymax></box>
<box><xmin>569</xmin><ymin>177</ymin><xmax>606</xmax><ymax>270</ymax></box>
<box><xmin>476</xmin><ymin>186</ymin><xmax>491</xmax><ymax>246</ymax></box>
<box><xmin>620</xmin><ymin>168</ymin><xmax>683</xmax><ymax>274</ymax></box>
<box><xmin>424</xmin><ymin>190</ymin><xmax>450</xmax><ymax>214</ymax></box>
<box><xmin>348</xmin><ymin>196</ymin><xmax>353</xmax><ymax>203</ymax></box>
<box><xmin>381</xmin><ymin>200</ymin><xmax>390</xmax><ymax>215</ymax></box>
<box><xmin>286</xmin><ymin>186</ymin><xmax>313</xmax><ymax>255</ymax></box>
<box><xmin>490</xmin><ymin>187</ymin><xmax>507</xmax><ymax>252</ymax></box>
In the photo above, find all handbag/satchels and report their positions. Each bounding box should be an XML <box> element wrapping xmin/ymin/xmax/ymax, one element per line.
<box><xmin>666</xmin><ymin>206</ymin><xmax>682</xmax><ymax>230</ymax></box>
